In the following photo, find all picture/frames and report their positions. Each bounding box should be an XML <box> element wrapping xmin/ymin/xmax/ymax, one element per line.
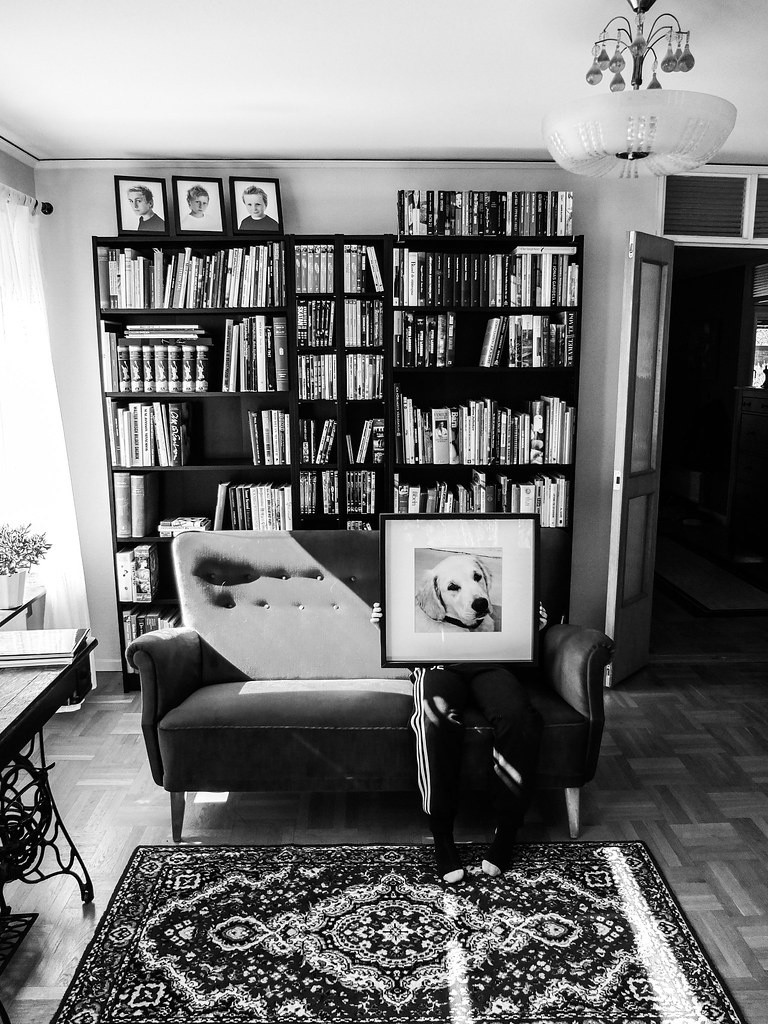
<box><xmin>114</xmin><ymin>175</ymin><xmax>170</xmax><ymax>236</ymax></box>
<box><xmin>171</xmin><ymin>175</ymin><xmax>228</xmax><ymax>236</ymax></box>
<box><xmin>379</xmin><ymin>511</ymin><xmax>541</xmax><ymax>668</ymax></box>
<box><xmin>229</xmin><ymin>176</ymin><xmax>285</xmax><ymax>235</ymax></box>
<box><xmin>687</xmin><ymin>318</ymin><xmax>720</xmax><ymax>381</ymax></box>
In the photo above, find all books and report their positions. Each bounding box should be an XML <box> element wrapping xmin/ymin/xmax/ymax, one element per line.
<box><xmin>116</xmin><ymin>546</ymin><xmax>180</xmax><ymax>673</ymax></box>
<box><xmin>0</xmin><ymin>628</ymin><xmax>88</xmax><ymax>668</ymax></box>
<box><xmin>97</xmin><ymin>243</ymin><xmax>384</xmax><ymax>539</ymax></box>
<box><xmin>396</xmin><ymin>191</ymin><xmax>580</xmax><ymax>528</ymax></box>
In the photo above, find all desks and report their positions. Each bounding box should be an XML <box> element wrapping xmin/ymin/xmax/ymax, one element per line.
<box><xmin>0</xmin><ymin>637</ymin><xmax>99</xmax><ymax>902</ymax></box>
<box><xmin>0</xmin><ymin>587</ymin><xmax>47</xmax><ymax>631</ymax></box>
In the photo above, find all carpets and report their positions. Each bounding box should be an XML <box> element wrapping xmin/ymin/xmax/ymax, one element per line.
<box><xmin>654</xmin><ymin>527</ymin><xmax>768</xmax><ymax>618</ymax></box>
<box><xmin>49</xmin><ymin>844</ymin><xmax>750</xmax><ymax>1024</ymax></box>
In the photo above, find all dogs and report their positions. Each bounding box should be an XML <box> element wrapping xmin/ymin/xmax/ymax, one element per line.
<box><xmin>416</xmin><ymin>553</ymin><xmax>495</xmax><ymax>632</ymax></box>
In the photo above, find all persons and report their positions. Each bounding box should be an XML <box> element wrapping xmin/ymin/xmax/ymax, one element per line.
<box><xmin>127</xmin><ymin>184</ymin><xmax>164</xmax><ymax>231</ymax></box>
<box><xmin>239</xmin><ymin>185</ymin><xmax>279</xmax><ymax>231</ymax></box>
<box><xmin>181</xmin><ymin>186</ymin><xmax>222</xmax><ymax>232</ymax></box>
<box><xmin>370</xmin><ymin>598</ymin><xmax>548</xmax><ymax>884</ymax></box>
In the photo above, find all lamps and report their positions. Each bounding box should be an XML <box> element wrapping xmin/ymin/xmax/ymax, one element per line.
<box><xmin>542</xmin><ymin>0</ymin><xmax>738</xmax><ymax>182</ymax></box>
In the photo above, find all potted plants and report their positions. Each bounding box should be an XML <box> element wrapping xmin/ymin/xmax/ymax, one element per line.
<box><xmin>0</xmin><ymin>522</ymin><xmax>53</xmax><ymax>610</ymax></box>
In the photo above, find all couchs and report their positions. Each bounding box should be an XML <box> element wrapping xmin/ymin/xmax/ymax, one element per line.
<box><xmin>121</xmin><ymin>529</ymin><xmax>619</xmax><ymax>840</ymax></box>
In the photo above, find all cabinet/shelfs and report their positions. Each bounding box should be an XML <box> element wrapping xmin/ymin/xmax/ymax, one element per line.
<box><xmin>91</xmin><ymin>234</ymin><xmax>585</xmax><ymax>696</ymax></box>
<box><xmin>723</xmin><ymin>385</ymin><xmax>768</xmax><ymax>559</ymax></box>
